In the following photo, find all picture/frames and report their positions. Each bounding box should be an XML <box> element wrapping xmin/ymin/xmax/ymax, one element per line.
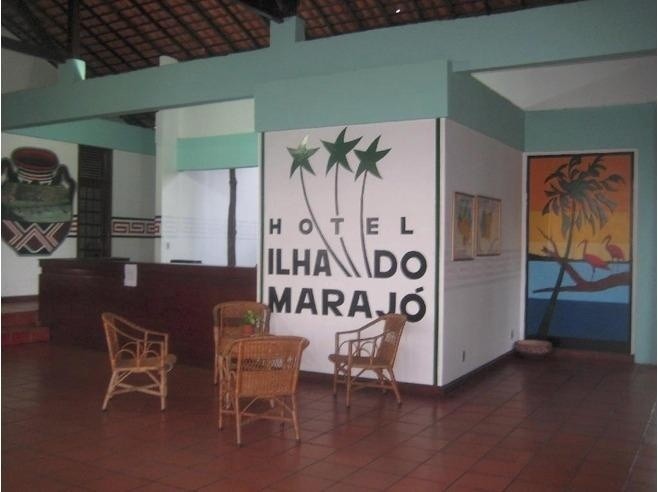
<box><xmin>451</xmin><ymin>191</ymin><xmax>503</xmax><ymax>261</ymax></box>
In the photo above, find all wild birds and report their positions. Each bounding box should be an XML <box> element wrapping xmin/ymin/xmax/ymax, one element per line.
<box><xmin>576</xmin><ymin>238</ymin><xmax>612</xmax><ymax>282</ymax></box>
<box><xmin>600</xmin><ymin>235</ymin><xmax>627</xmax><ymax>273</ymax></box>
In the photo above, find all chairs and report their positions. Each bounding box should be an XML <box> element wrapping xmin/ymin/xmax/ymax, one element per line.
<box><xmin>99</xmin><ymin>311</ymin><xmax>177</xmax><ymax>413</ymax></box>
<box><xmin>212</xmin><ymin>300</ymin><xmax>310</xmax><ymax>446</ymax></box>
<box><xmin>328</xmin><ymin>311</ymin><xmax>408</xmax><ymax>411</ymax></box>
